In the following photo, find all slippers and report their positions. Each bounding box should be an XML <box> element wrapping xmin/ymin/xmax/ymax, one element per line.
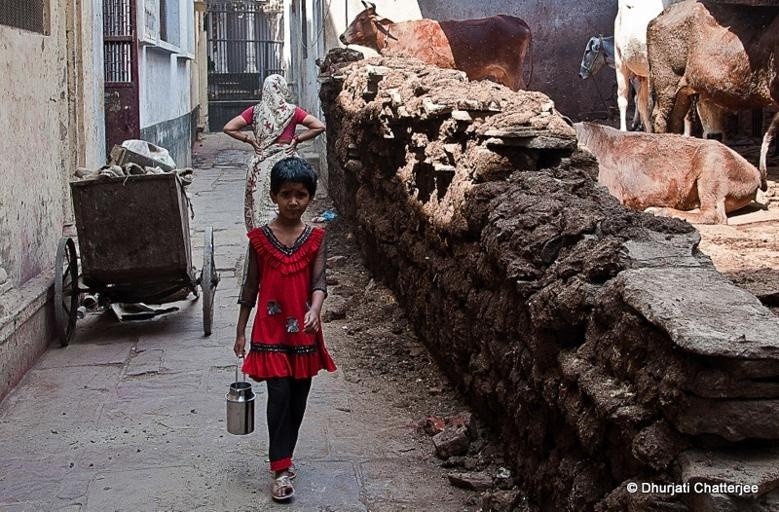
<box><xmin>271</xmin><ymin>461</ymin><xmax>296</xmax><ymax>501</ymax></box>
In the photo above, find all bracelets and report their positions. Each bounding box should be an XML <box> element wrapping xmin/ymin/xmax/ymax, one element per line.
<box><xmin>294</xmin><ymin>136</ymin><xmax>300</xmax><ymax>143</ymax></box>
<box><xmin>244</xmin><ymin>134</ymin><xmax>248</xmax><ymax>141</ymax></box>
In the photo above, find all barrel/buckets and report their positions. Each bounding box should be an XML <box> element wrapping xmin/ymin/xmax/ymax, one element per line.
<box><xmin>225</xmin><ymin>354</ymin><xmax>256</xmax><ymax>435</ymax></box>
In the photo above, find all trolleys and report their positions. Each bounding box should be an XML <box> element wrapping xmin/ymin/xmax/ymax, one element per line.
<box><xmin>53</xmin><ymin>168</ymin><xmax>216</xmax><ymax>347</ymax></box>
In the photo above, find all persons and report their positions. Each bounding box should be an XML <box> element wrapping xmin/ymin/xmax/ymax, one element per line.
<box><xmin>223</xmin><ymin>73</ymin><xmax>326</xmax><ymax>237</ymax></box>
<box><xmin>232</xmin><ymin>156</ymin><xmax>336</xmax><ymax>501</ymax></box>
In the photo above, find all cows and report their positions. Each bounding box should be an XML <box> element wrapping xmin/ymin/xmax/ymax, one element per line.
<box><xmin>614</xmin><ymin>0</ymin><xmax>692</xmax><ymax>136</ymax></box>
<box><xmin>578</xmin><ymin>32</ymin><xmax>644</xmax><ymax>132</ymax></box>
<box><xmin>646</xmin><ymin>1</ymin><xmax>779</xmax><ymax>143</ymax></box>
<box><xmin>554</xmin><ymin>108</ymin><xmax>779</xmax><ymax>226</ymax></box>
<box><xmin>338</xmin><ymin>0</ymin><xmax>533</xmax><ymax>93</ymax></box>
